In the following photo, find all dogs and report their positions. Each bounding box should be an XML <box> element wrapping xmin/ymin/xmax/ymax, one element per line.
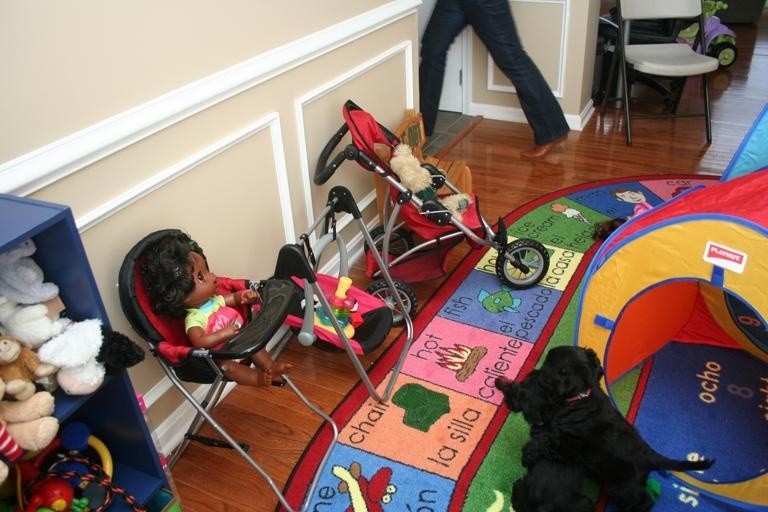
<box><xmin>494</xmin><ymin>344</ymin><xmax>717</xmax><ymax>512</ymax></box>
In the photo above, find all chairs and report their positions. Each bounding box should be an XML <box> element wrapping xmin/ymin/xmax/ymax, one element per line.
<box><xmin>600</xmin><ymin>0</ymin><xmax>719</xmax><ymax>146</ymax></box>
<box><xmin>120</xmin><ymin>226</ymin><xmax>338</xmax><ymax>511</ymax></box>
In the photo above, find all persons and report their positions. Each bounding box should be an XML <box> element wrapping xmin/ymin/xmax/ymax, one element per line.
<box><xmin>419</xmin><ymin>0</ymin><xmax>570</xmax><ymax>159</ymax></box>
<box><xmin>143</xmin><ymin>233</ymin><xmax>293</xmax><ymax>387</ymax></box>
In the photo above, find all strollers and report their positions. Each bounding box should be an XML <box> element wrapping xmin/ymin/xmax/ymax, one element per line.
<box><xmin>313</xmin><ymin>100</ymin><xmax>550</xmax><ymax>325</ymax></box>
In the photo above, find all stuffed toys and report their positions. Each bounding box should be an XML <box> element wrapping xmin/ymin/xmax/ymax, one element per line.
<box><xmin>389</xmin><ymin>142</ymin><xmax>473</xmax><ymax>223</ymax></box>
<box><xmin>0</xmin><ymin>237</ymin><xmax>147</xmax><ymax>512</ymax></box>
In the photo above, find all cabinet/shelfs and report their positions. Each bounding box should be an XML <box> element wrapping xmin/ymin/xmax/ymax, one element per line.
<box><xmin>1</xmin><ymin>192</ymin><xmax>182</xmax><ymax>511</ymax></box>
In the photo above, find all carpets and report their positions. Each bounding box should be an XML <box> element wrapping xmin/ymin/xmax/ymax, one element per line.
<box><xmin>269</xmin><ymin>173</ymin><xmax>765</xmax><ymax>512</ymax></box>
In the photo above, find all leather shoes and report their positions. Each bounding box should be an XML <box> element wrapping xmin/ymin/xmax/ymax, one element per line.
<box><xmin>520</xmin><ymin>133</ymin><xmax>567</xmax><ymax>159</ymax></box>
